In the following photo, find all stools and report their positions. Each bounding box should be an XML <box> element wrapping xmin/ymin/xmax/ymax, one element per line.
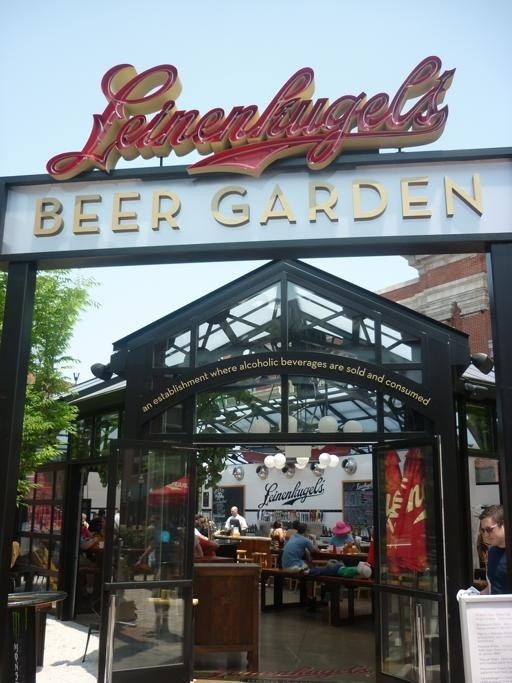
<box><xmin>236</xmin><ymin>550</ymin><xmax>279</xmax><ymax>587</ymax></box>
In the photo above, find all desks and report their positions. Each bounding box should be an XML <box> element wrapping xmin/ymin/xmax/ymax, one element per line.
<box><xmin>214</xmin><ymin>536</ymin><xmax>272</xmax><ymax>568</ymax></box>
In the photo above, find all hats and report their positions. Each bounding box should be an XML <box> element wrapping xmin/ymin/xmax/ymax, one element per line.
<box><xmin>331</xmin><ymin>521</ymin><xmax>351</xmax><ymax>535</ymax></box>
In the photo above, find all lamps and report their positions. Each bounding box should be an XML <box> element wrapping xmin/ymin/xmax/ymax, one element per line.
<box><xmin>251</xmin><ymin>377</ymin><xmax>364</xmax><ymax>471</ymax></box>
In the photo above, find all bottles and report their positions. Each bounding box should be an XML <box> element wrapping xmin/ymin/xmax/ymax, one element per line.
<box><xmin>262</xmin><ymin>509</ymin><xmax>322</xmax><ymax>523</ymax></box>
<box><xmin>246</xmin><ymin>523</ymin><xmax>257</xmax><ymax>533</ymax></box>
<box><xmin>321</xmin><ymin>526</ymin><xmax>331</xmax><ymax>537</ymax></box>
<box><xmin>332</xmin><ymin>541</ymin><xmax>357</xmax><ymax>554</ymax></box>
<box><xmin>231</xmin><ymin>524</ymin><xmax>240</xmax><ymax>536</ymax></box>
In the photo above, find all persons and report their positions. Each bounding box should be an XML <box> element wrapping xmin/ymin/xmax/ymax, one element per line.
<box><xmin>285</xmin><ymin>520</ymin><xmax>299</xmax><ymax>541</ymax></box>
<box><xmin>269</xmin><ymin>521</ymin><xmax>283</xmax><ymax>554</ymax></box>
<box><xmin>80</xmin><ymin>504</ymin><xmax>248</xmax><ymax>612</ymax></box>
<box><xmin>10</xmin><ymin>505</ymin><xmax>20</xmax><ymax>570</ymax></box>
<box><xmin>320</xmin><ymin>520</ymin><xmax>353</xmax><ymax>603</ymax></box>
<box><xmin>455</xmin><ymin>504</ymin><xmax>508</xmax><ymax>601</ymax></box>
<box><xmin>281</xmin><ymin>523</ymin><xmax>317</xmax><ymax>612</ymax></box>
<box><xmin>291</xmin><ymin>560</ymin><xmax>372</xmax><ymax>578</ymax></box>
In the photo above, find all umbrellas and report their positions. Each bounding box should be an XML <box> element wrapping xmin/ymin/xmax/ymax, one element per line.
<box><xmin>385</xmin><ymin>447</ymin><xmax>434</xmax><ymax>664</ymax></box>
<box><xmin>368</xmin><ymin>448</ymin><xmax>407</xmax><ymax>646</ymax></box>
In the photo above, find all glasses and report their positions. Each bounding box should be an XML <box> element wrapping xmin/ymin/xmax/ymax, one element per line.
<box><xmin>480</xmin><ymin>525</ymin><xmax>497</xmax><ymax>534</ymax></box>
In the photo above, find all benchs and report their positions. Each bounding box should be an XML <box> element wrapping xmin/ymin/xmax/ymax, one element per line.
<box><xmin>261</xmin><ymin>568</ymin><xmax>374</xmax><ymax>627</ymax></box>
